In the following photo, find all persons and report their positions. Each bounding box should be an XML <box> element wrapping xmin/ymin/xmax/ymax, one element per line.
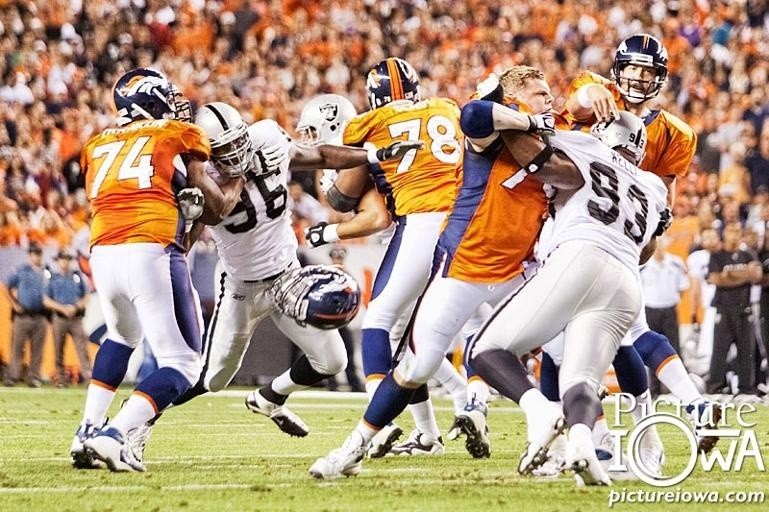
<box><xmin>3</xmin><ymin>245</ymin><xmax>52</xmax><ymax>388</ymax></box>
<box><xmin>705</xmin><ymin>220</ymin><xmax>765</xmax><ymax>397</ymax></box>
<box><xmin>468</xmin><ymin>111</ymin><xmax>674</xmax><ymax>486</ymax></box>
<box><xmin>1</xmin><ymin>1</ymin><xmax>119</xmax><ymax>246</ymax></box>
<box><xmin>662</xmin><ymin>0</ymin><xmax>768</xmax><ymax>232</ymax></box>
<box><xmin>295</xmin><ymin>94</ymin><xmax>467</xmax><ymax>458</ymax></box>
<box><xmin>325</xmin><ymin>57</ymin><xmax>493</xmax><ymax>459</ymax></box>
<box><xmin>638</xmin><ymin>234</ymin><xmax>690</xmax><ymax>404</ymax></box>
<box><xmin>287</xmin><ymin>180</ymin><xmax>329</xmax><ymax>226</ymax></box>
<box><xmin>307</xmin><ymin>65</ymin><xmax>659</xmax><ymax>483</ymax></box>
<box><xmin>41</xmin><ymin>251</ymin><xmax>93</xmax><ymax>389</ymax></box>
<box><xmin>69</xmin><ymin>67</ymin><xmax>286</xmax><ymax>471</ymax></box>
<box><xmin>565</xmin><ymin>34</ymin><xmax>697</xmax><ymax>482</ymax></box>
<box><xmin>118</xmin><ymin>1</ymin><xmax>661</xmax><ymax>138</ymax></box>
<box><xmin>475</xmin><ymin>73</ymin><xmax>722</xmax><ymax>453</ymax></box>
<box><xmin>740</xmin><ymin>227</ymin><xmax>769</xmax><ymax>394</ymax></box>
<box><xmin>119</xmin><ymin>101</ymin><xmax>425</xmax><ymax>471</ymax></box>
<box><xmin>686</xmin><ymin>227</ymin><xmax>739</xmax><ymax>396</ymax></box>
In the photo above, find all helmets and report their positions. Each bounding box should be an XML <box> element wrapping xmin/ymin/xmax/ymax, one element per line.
<box><xmin>610</xmin><ymin>33</ymin><xmax>670</xmax><ymax>105</ymax></box>
<box><xmin>194</xmin><ymin>101</ymin><xmax>256</xmax><ymax>178</ymax></box>
<box><xmin>590</xmin><ymin>109</ymin><xmax>648</xmax><ymax>168</ymax></box>
<box><xmin>295</xmin><ymin>94</ymin><xmax>357</xmax><ymax>147</ymax></box>
<box><xmin>365</xmin><ymin>57</ymin><xmax>421</xmax><ymax>111</ymax></box>
<box><xmin>114</xmin><ymin>69</ymin><xmax>178</xmax><ymax>126</ymax></box>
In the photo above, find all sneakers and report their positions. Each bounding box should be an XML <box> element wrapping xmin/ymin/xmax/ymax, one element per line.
<box><xmin>127</xmin><ymin>425</ymin><xmax>153</xmax><ymax>472</ymax></box>
<box><xmin>446</xmin><ymin>415</ymin><xmax>462</xmax><ymax>440</ymax></box>
<box><xmin>517</xmin><ymin>402</ymin><xmax>568</xmax><ymax>475</ymax></box>
<box><xmin>246</xmin><ymin>387</ymin><xmax>309</xmax><ymax>437</ymax></box>
<box><xmin>454</xmin><ymin>393</ymin><xmax>493</xmax><ymax>458</ymax></box>
<box><xmin>82</xmin><ymin>425</ymin><xmax>133</xmax><ymax>472</ymax></box>
<box><xmin>640</xmin><ymin>440</ymin><xmax>667</xmax><ymax>476</ymax></box>
<box><xmin>308</xmin><ymin>448</ymin><xmax>364</xmax><ymax>481</ymax></box>
<box><xmin>386</xmin><ymin>428</ymin><xmax>446</xmax><ymax>456</ymax></box>
<box><xmin>365</xmin><ymin>420</ymin><xmax>404</xmax><ymax>458</ymax></box>
<box><xmin>530</xmin><ymin>453</ymin><xmax>566</xmax><ymax>476</ymax></box>
<box><xmin>70</xmin><ymin>423</ymin><xmax>102</xmax><ymax>469</ymax></box>
<box><xmin>595</xmin><ymin>433</ymin><xmax>615</xmax><ymax>471</ymax></box>
<box><xmin>686</xmin><ymin>402</ymin><xmax>722</xmax><ymax>454</ymax></box>
<box><xmin>564</xmin><ymin>440</ymin><xmax>614</xmax><ymax>488</ymax></box>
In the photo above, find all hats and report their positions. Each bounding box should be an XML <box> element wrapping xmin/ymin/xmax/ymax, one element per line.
<box><xmin>269</xmin><ymin>263</ymin><xmax>361</xmax><ymax>330</ymax></box>
<box><xmin>29</xmin><ymin>242</ymin><xmax>43</xmax><ymax>252</ymax></box>
<box><xmin>58</xmin><ymin>248</ymin><xmax>74</xmax><ymax>260</ymax></box>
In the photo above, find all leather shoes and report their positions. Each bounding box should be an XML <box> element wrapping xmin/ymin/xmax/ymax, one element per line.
<box><xmin>4</xmin><ymin>377</ymin><xmax>17</xmax><ymax>386</ymax></box>
<box><xmin>28</xmin><ymin>378</ymin><xmax>42</xmax><ymax>388</ymax></box>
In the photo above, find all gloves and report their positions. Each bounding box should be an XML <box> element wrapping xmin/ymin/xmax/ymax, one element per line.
<box><xmin>241</xmin><ymin>141</ymin><xmax>287</xmax><ymax>182</ymax></box>
<box><xmin>177</xmin><ymin>187</ymin><xmax>205</xmax><ymax>233</ymax></box>
<box><xmin>655</xmin><ymin>208</ymin><xmax>674</xmax><ymax>236</ymax></box>
<box><xmin>367</xmin><ymin>141</ymin><xmax>425</xmax><ymax>164</ymax></box>
<box><xmin>477</xmin><ymin>73</ymin><xmax>503</xmax><ymax>105</ymax></box>
<box><xmin>528</xmin><ymin>110</ymin><xmax>557</xmax><ymax>136</ymax></box>
<box><xmin>304</xmin><ymin>222</ymin><xmax>341</xmax><ymax>249</ymax></box>
